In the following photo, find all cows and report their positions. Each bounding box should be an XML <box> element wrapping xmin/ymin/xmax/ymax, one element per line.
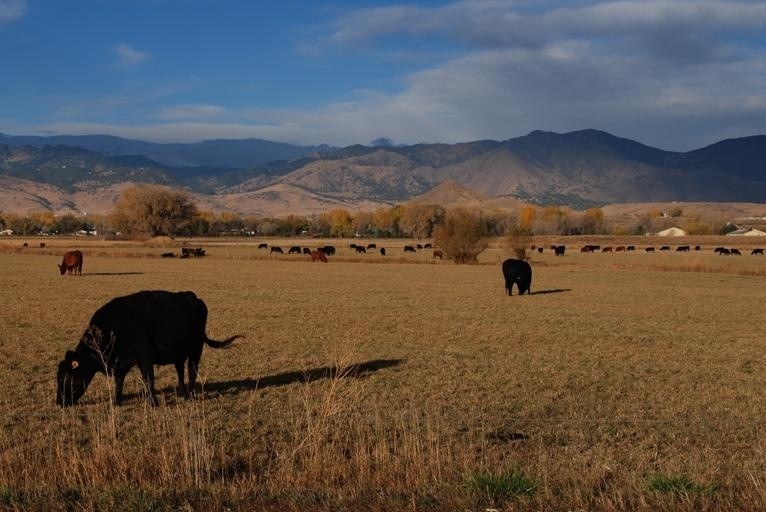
<box><xmin>258</xmin><ymin>244</ymin><xmax>267</xmax><ymax>248</ymax></box>
<box><xmin>503</xmin><ymin>258</ymin><xmax>532</xmax><ymax>296</ymax></box>
<box><xmin>350</xmin><ymin>243</ymin><xmax>432</xmax><ymax>256</ymax></box>
<box><xmin>288</xmin><ymin>246</ymin><xmax>336</xmax><ymax>264</ymax></box>
<box><xmin>161</xmin><ymin>247</ymin><xmax>206</xmax><ymax>258</ymax></box>
<box><xmin>271</xmin><ymin>247</ymin><xmax>283</xmax><ymax>254</ymax></box>
<box><xmin>538</xmin><ymin>243</ymin><xmax>765</xmax><ymax>257</ymax></box>
<box><xmin>433</xmin><ymin>250</ymin><xmax>443</xmax><ymax>259</ymax></box>
<box><xmin>56</xmin><ymin>291</ymin><xmax>247</xmax><ymax>408</ymax></box>
<box><xmin>58</xmin><ymin>250</ymin><xmax>83</xmax><ymax>276</ymax></box>
